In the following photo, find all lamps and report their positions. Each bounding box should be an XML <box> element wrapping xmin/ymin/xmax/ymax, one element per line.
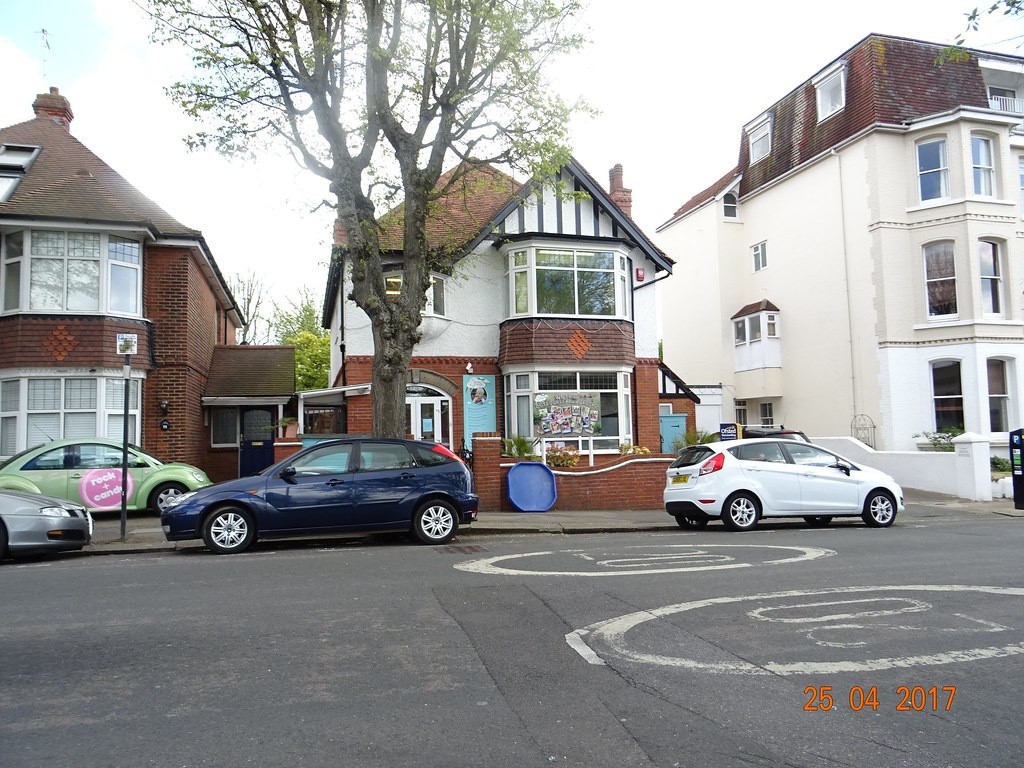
<box><xmin>159</xmin><ymin>399</ymin><xmax>169</xmax><ymax>415</ymax></box>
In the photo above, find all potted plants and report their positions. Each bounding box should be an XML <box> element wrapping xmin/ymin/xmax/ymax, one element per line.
<box><xmin>281</xmin><ymin>418</ymin><xmax>299</xmax><ymax>438</ymax></box>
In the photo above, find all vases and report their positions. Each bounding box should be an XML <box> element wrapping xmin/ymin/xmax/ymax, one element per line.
<box><xmin>554</xmin><ymin>463</ymin><xmax>560</xmax><ymax>467</ymax></box>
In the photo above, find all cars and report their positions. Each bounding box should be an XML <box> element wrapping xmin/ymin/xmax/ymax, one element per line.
<box><xmin>660</xmin><ymin>435</ymin><xmax>905</xmax><ymax>532</ymax></box>
<box><xmin>159</xmin><ymin>436</ymin><xmax>480</xmax><ymax>556</ymax></box>
<box><xmin>0</xmin><ymin>488</ymin><xmax>95</xmax><ymax>559</ymax></box>
<box><xmin>0</xmin><ymin>436</ymin><xmax>216</xmax><ymax>517</ymax></box>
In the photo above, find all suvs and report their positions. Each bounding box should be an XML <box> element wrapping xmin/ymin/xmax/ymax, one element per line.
<box><xmin>703</xmin><ymin>423</ymin><xmax>817</xmax><ymax>463</ymax></box>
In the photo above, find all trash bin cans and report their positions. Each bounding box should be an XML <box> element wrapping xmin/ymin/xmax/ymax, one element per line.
<box><xmin>296</xmin><ymin>434</ymin><xmax>368</xmax><ymax>450</ymax></box>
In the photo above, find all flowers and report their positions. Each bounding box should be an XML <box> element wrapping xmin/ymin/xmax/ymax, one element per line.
<box><xmin>546</xmin><ymin>442</ymin><xmax>580</xmax><ymax>467</ymax></box>
